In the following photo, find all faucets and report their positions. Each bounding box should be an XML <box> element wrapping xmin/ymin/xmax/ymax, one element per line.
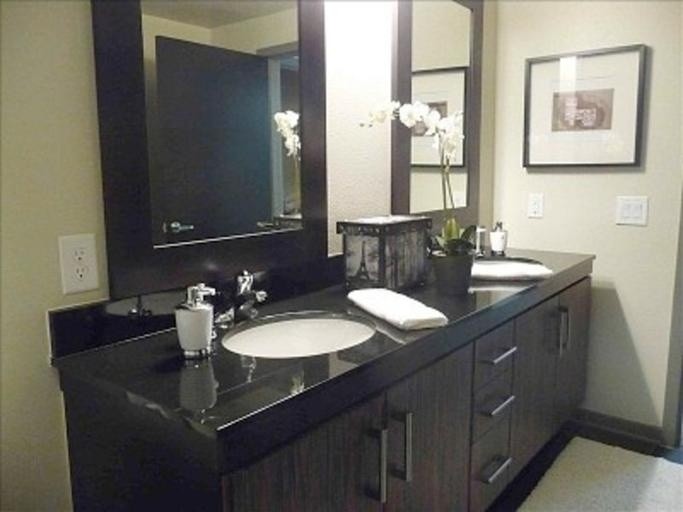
<box><xmin>213</xmin><ymin>270</ymin><xmax>267</xmax><ymax>324</ymax></box>
<box><xmin>129</xmin><ymin>296</ymin><xmax>152</xmax><ymax>321</ymax></box>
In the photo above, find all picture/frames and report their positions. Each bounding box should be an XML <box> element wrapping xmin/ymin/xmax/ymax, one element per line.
<box><xmin>523</xmin><ymin>44</ymin><xmax>647</xmax><ymax>168</ymax></box>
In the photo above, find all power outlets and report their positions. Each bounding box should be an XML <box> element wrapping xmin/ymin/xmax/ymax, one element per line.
<box><xmin>528</xmin><ymin>193</ymin><xmax>542</xmax><ymax>218</ymax></box>
<box><xmin>57</xmin><ymin>233</ymin><xmax>100</xmax><ymax>297</ymax></box>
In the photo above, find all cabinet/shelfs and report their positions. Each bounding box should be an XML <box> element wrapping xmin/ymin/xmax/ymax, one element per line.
<box><xmin>54</xmin><ymin>317</ymin><xmax>471</xmax><ymax>512</ymax></box>
<box><xmin>517</xmin><ymin>255</ymin><xmax>592</xmax><ymax>504</ymax></box>
<box><xmin>468</xmin><ymin>295</ymin><xmax>517</xmax><ymax>511</ymax></box>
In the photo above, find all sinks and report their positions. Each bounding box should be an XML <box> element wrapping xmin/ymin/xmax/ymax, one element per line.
<box><xmin>222</xmin><ymin>310</ymin><xmax>376</xmax><ymax>359</ymax></box>
<box><xmin>106</xmin><ymin>292</ymin><xmax>186</xmax><ymax>315</ymax></box>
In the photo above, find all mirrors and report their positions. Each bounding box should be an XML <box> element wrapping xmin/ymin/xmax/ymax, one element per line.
<box><xmin>90</xmin><ymin>0</ymin><xmax>328</xmax><ymax>301</ymax></box>
<box><xmin>390</xmin><ymin>0</ymin><xmax>482</xmax><ymax>228</ymax></box>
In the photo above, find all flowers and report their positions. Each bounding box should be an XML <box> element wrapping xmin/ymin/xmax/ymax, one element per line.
<box><xmin>274</xmin><ymin>111</ymin><xmax>302</xmax><ymax>161</ymax></box>
<box><xmin>385</xmin><ymin>102</ymin><xmax>476</xmax><ymax>252</ymax></box>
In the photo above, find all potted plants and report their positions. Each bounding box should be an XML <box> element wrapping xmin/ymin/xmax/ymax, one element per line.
<box><xmin>431</xmin><ymin>216</ymin><xmax>476</xmax><ymax>294</ymax></box>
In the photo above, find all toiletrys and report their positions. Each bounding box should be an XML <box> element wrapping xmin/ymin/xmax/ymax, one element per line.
<box><xmin>489</xmin><ymin>221</ymin><xmax>508</xmax><ymax>255</ymax></box>
<box><xmin>175</xmin><ymin>284</ymin><xmax>216</xmax><ymax>358</ymax></box>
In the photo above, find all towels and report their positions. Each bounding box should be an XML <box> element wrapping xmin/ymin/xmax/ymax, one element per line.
<box><xmin>473</xmin><ymin>260</ymin><xmax>552</xmax><ymax>283</ymax></box>
<box><xmin>347</xmin><ymin>287</ymin><xmax>448</xmax><ymax>330</ymax></box>
